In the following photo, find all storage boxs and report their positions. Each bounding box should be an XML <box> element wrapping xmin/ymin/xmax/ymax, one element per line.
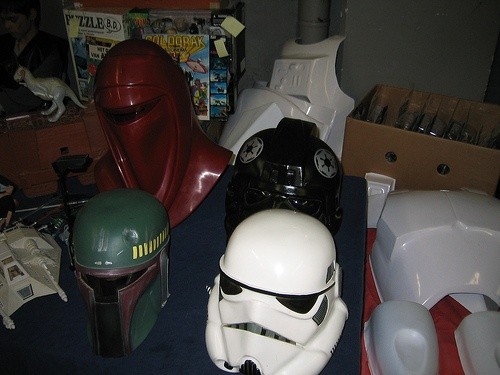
<box><xmin>340</xmin><ymin>85</ymin><xmax>500</xmax><ymax>194</ymax></box>
<box><xmin>61</xmin><ymin>0</ymin><xmax>245</xmax><ymax>124</ymax></box>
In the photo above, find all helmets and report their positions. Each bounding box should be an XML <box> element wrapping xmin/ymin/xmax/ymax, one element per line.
<box><xmin>69</xmin><ymin>188</ymin><xmax>172</xmax><ymax>357</ymax></box>
<box><xmin>91</xmin><ymin>39</ymin><xmax>233</xmax><ymax>229</ymax></box>
<box><xmin>224</xmin><ymin>117</ymin><xmax>344</xmax><ymax>246</ymax></box>
<box><xmin>204</xmin><ymin>210</ymin><xmax>348</xmax><ymax>375</ymax></box>
